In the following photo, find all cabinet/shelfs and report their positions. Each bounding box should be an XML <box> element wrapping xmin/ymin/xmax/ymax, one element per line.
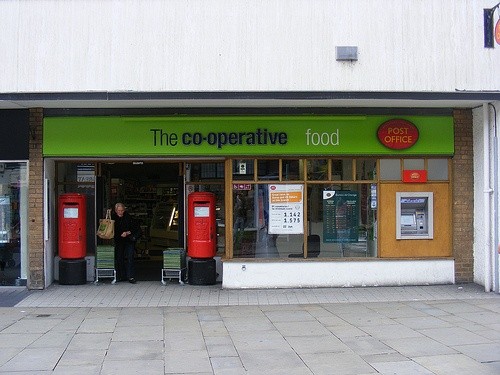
<box><xmin>122</xmin><ymin>192</ymin><xmax>180</xmax><ymax>256</ymax></box>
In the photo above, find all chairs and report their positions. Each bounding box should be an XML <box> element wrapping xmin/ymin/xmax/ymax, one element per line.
<box><xmin>288</xmin><ymin>235</ymin><xmax>320</xmax><ymax>257</ymax></box>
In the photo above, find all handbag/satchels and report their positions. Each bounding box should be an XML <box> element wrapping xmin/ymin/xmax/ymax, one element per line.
<box><xmin>126</xmin><ymin>222</ymin><xmax>143</xmax><ymax>243</ymax></box>
<box><xmin>96</xmin><ymin>208</ymin><xmax>115</xmax><ymax>240</ymax></box>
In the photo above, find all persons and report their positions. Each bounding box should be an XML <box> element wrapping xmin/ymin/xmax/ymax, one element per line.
<box><xmin>107</xmin><ymin>203</ymin><xmax>138</xmax><ymax>283</ymax></box>
<box><xmin>233</xmin><ymin>194</ymin><xmax>247</xmax><ymax>249</ymax></box>
